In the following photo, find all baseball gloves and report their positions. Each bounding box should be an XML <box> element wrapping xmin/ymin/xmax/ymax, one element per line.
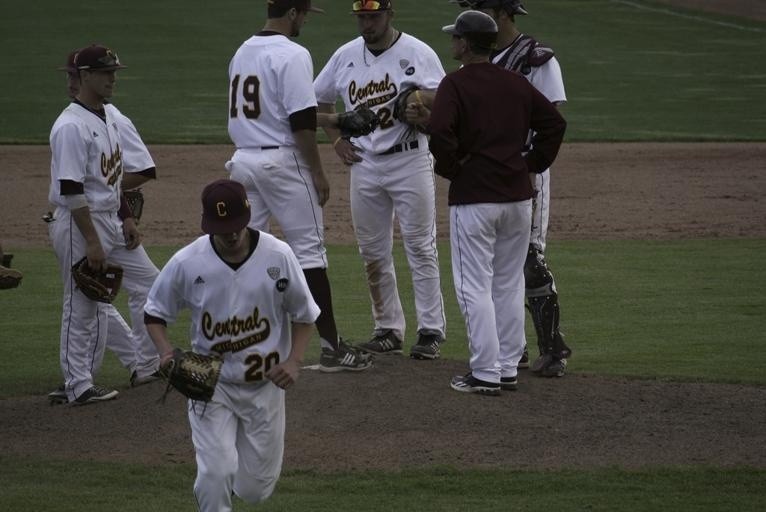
<box><xmin>395</xmin><ymin>88</ymin><xmax>438</xmax><ymax>133</ymax></box>
<box><xmin>124</xmin><ymin>190</ymin><xmax>144</xmax><ymax>223</ymax></box>
<box><xmin>336</xmin><ymin>107</ymin><xmax>379</xmax><ymax>140</ymax></box>
<box><xmin>160</xmin><ymin>348</ymin><xmax>223</xmax><ymax>403</ymax></box>
<box><xmin>72</xmin><ymin>258</ymin><xmax>123</xmax><ymax>304</ymax></box>
<box><xmin>0</xmin><ymin>253</ymin><xmax>22</xmax><ymax>289</ymax></box>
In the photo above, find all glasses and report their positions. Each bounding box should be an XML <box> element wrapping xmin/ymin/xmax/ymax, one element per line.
<box><xmin>351</xmin><ymin>1</ymin><xmax>390</xmax><ymax>12</ymax></box>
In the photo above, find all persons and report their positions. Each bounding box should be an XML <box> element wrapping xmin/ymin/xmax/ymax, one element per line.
<box><xmin>404</xmin><ymin>1</ymin><xmax>572</xmax><ymax>378</ymax></box>
<box><xmin>427</xmin><ymin>9</ymin><xmax>568</xmax><ymax>396</ymax></box>
<box><xmin>225</xmin><ymin>1</ymin><xmax>377</xmax><ymax>372</ymax></box>
<box><xmin>140</xmin><ymin>178</ymin><xmax>324</xmax><ymax>510</ymax></box>
<box><xmin>311</xmin><ymin>2</ymin><xmax>449</xmax><ymax>361</ymax></box>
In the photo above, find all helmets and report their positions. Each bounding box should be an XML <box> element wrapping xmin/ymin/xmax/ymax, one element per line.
<box><xmin>440</xmin><ymin>10</ymin><xmax>501</xmax><ymax>50</ymax></box>
<box><xmin>460</xmin><ymin>1</ymin><xmax>528</xmax><ymax>49</ymax></box>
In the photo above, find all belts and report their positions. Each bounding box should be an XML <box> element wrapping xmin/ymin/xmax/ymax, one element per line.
<box><xmin>375</xmin><ymin>140</ymin><xmax>418</xmax><ymax>155</ymax></box>
<box><xmin>261</xmin><ymin>145</ymin><xmax>279</xmax><ymax>149</ymax></box>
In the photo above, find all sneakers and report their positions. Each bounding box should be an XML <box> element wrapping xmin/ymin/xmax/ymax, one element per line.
<box><xmin>452</xmin><ymin>371</ymin><xmax>503</xmax><ymax>394</ymax></box>
<box><xmin>129</xmin><ymin>370</ymin><xmax>162</xmax><ymax>391</ymax></box>
<box><xmin>319</xmin><ymin>336</ymin><xmax>373</xmax><ymax>373</ymax></box>
<box><xmin>501</xmin><ymin>376</ymin><xmax>518</xmax><ymax>389</ymax></box>
<box><xmin>518</xmin><ymin>344</ymin><xmax>531</xmax><ymax>367</ymax></box>
<box><xmin>355</xmin><ymin>330</ymin><xmax>405</xmax><ymax>355</ymax></box>
<box><xmin>540</xmin><ymin>354</ymin><xmax>567</xmax><ymax>378</ymax></box>
<box><xmin>72</xmin><ymin>387</ymin><xmax>119</xmax><ymax>405</ymax></box>
<box><xmin>409</xmin><ymin>328</ymin><xmax>443</xmax><ymax>359</ymax></box>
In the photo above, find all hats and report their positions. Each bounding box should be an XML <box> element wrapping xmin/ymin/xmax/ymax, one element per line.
<box><xmin>200</xmin><ymin>179</ymin><xmax>252</xmax><ymax>234</ymax></box>
<box><xmin>59</xmin><ymin>50</ymin><xmax>80</xmax><ymax>74</ymax></box>
<box><xmin>268</xmin><ymin>0</ymin><xmax>326</xmax><ymax>13</ymax></box>
<box><xmin>348</xmin><ymin>1</ymin><xmax>395</xmax><ymax>15</ymax></box>
<box><xmin>75</xmin><ymin>46</ymin><xmax>129</xmax><ymax>74</ymax></box>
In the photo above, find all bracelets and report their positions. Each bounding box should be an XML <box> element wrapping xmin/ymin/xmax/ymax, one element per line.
<box><xmin>332</xmin><ymin>136</ymin><xmax>341</xmax><ymax>148</ymax></box>
<box><xmin>413</xmin><ymin>89</ymin><xmax>420</xmax><ymax>104</ymax></box>
